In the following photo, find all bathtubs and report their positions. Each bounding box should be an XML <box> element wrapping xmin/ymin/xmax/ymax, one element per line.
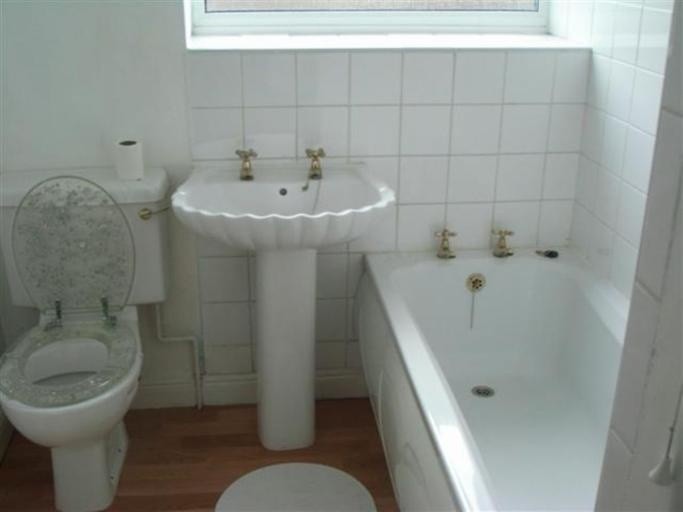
<box><xmin>364</xmin><ymin>245</ymin><xmax>629</xmax><ymax>510</ymax></box>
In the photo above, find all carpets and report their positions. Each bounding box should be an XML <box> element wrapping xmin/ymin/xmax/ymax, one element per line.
<box><xmin>212</xmin><ymin>462</ymin><xmax>377</xmax><ymax>511</ymax></box>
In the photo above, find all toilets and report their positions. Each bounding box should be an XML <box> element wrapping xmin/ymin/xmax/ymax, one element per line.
<box><xmin>0</xmin><ymin>162</ymin><xmax>170</xmax><ymax>508</ymax></box>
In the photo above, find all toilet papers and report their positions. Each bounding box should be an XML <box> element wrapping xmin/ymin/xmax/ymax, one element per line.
<box><xmin>114</xmin><ymin>136</ymin><xmax>145</xmax><ymax>183</ymax></box>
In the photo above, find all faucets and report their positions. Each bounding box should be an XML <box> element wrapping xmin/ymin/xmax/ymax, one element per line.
<box><xmin>234</xmin><ymin>149</ymin><xmax>258</xmax><ymax>181</ymax></box>
<box><xmin>433</xmin><ymin>228</ymin><xmax>458</xmax><ymax>260</ymax></box>
<box><xmin>305</xmin><ymin>146</ymin><xmax>326</xmax><ymax>181</ymax></box>
<box><xmin>491</xmin><ymin>228</ymin><xmax>516</xmax><ymax>258</ymax></box>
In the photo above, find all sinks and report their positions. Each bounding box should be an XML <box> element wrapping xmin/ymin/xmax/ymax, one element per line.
<box><xmin>172</xmin><ymin>176</ymin><xmax>394</xmax><ymax>249</ymax></box>
